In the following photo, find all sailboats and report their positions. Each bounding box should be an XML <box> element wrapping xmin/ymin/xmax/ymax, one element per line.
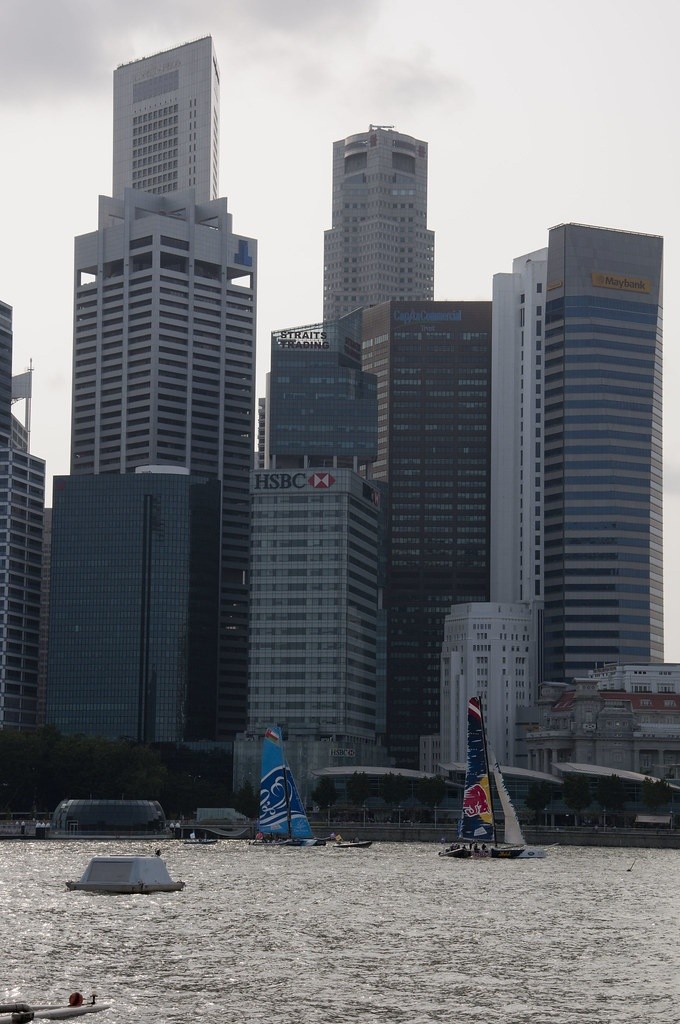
<box><xmin>244</xmin><ymin>725</ymin><xmax>326</xmax><ymax>847</ymax></box>
<box><xmin>437</xmin><ymin>695</ymin><xmax>559</xmax><ymax>859</ymax></box>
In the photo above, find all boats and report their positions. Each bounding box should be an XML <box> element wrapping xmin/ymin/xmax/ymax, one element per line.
<box><xmin>333</xmin><ymin>840</ymin><xmax>372</xmax><ymax>850</ymax></box>
<box><xmin>183</xmin><ymin>839</ymin><xmax>218</xmax><ymax>845</ymax></box>
<box><xmin>64</xmin><ymin>848</ymin><xmax>188</xmax><ymax>893</ymax></box>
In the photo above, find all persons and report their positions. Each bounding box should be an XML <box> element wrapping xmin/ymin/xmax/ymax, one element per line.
<box><xmin>445</xmin><ymin>841</ymin><xmax>486</xmax><ymax>853</ymax></box>
